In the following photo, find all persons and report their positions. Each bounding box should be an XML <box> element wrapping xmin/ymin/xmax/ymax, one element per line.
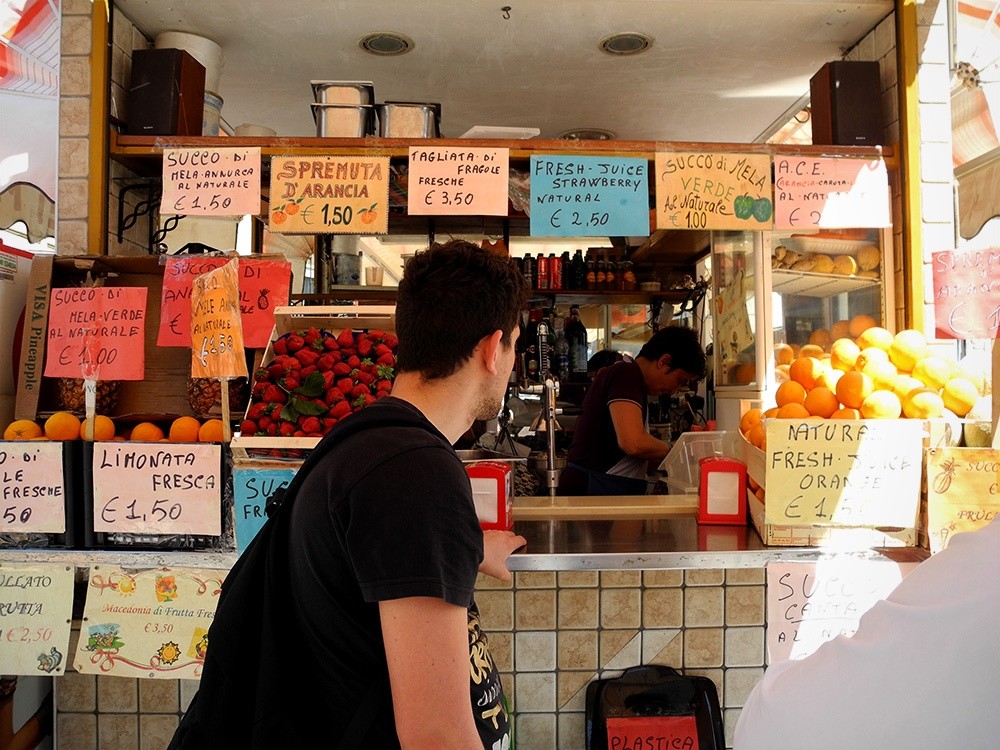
<box><xmin>558</xmin><ymin>323</ymin><xmax>707</xmax><ymax>496</ymax></box>
<box><xmin>256</xmin><ymin>235</ymin><xmax>528</xmax><ymax>750</ymax></box>
<box><xmin>732</xmin><ymin>512</ymin><xmax>1000</xmax><ymax>750</ymax></box>
<box><xmin>587</xmin><ymin>350</ymin><xmax>623</xmax><ymax>378</ymax></box>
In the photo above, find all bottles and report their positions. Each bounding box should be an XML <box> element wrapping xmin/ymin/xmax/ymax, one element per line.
<box><xmin>526</xmin><ymin>250</ymin><xmax>636</xmax><ymax>291</ymax></box>
<box><xmin>520</xmin><ymin>303</ymin><xmax>589</xmax><ymax>381</ymax></box>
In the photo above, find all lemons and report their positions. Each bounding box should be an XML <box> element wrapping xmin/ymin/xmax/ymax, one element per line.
<box><xmin>771</xmin><ymin>246</ymin><xmax>882</xmax><ymax>278</ymax></box>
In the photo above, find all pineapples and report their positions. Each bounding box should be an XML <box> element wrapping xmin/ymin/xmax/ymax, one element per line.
<box><xmin>57</xmin><ymin>376</ymin><xmax>121</xmax><ymax>415</ymax></box>
<box><xmin>187</xmin><ymin>363</ymin><xmax>242</xmax><ymax>415</ymax></box>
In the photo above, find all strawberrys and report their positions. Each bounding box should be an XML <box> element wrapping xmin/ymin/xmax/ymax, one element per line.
<box><xmin>241</xmin><ymin>326</ymin><xmax>398</xmax><ymax>458</ymax></box>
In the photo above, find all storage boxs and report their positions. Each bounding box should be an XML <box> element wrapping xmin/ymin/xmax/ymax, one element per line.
<box><xmin>733</xmin><ymin>391</ymin><xmax>921</xmax><ymax>551</ymax></box>
<box><xmin>658</xmin><ymin>424</ymin><xmax>730</xmax><ymax>498</ymax></box>
<box><xmin>14</xmin><ymin>253</ymin><xmax>396</xmax><ymax>461</ymax></box>
<box><xmin>303</xmin><ymin>73</ymin><xmax>444</xmax><ymax>140</ymax></box>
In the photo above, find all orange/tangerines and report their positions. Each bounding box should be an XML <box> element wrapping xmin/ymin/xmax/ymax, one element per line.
<box><xmin>734</xmin><ymin>315</ymin><xmax>981</xmax><ymax>503</ymax></box>
<box><xmin>3</xmin><ymin>415</ymin><xmax>225</xmax><ymax>444</ymax></box>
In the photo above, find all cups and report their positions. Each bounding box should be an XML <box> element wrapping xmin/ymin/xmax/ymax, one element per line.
<box><xmin>649</xmin><ymin>422</ymin><xmax>671</xmax><ymax>444</ymax></box>
<box><xmin>365</xmin><ymin>266</ymin><xmax>382</xmax><ymax>285</ymax></box>
<box><xmin>333</xmin><ymin>252</ymin><xmax>362</xmax><ymax>286</ymax></box>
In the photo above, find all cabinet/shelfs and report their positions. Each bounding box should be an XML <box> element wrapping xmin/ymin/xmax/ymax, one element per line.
<box><xmin>103</xmin><ymin>127</ymin><xmax>901</xmax><ymax>525</ymax></box>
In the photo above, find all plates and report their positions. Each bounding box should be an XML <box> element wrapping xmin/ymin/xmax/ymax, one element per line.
<box><xmin>780</xmin><ymin>236</ymin><xmax>876</xmax><ymax>254</ymax></box>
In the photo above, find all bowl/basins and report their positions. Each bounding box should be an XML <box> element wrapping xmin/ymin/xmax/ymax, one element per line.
<box><xmin>640</xmin><ymin>283</ymin><xmax>661</xmax><ymax>291</ymax></box>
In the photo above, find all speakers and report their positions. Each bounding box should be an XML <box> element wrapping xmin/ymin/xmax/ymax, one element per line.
<box><xmin>127</xmin><ymin>47</ymin><xmax>208</xmax><ymax>135</ymax></box>
<box><xmin>809</xmin><ymin>58</ymin><xmax>900</xmax><ymax>151</ymax></box>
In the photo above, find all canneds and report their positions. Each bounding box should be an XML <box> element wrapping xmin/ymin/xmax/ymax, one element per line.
<box><xmin>510</xmin><ymin>256</ymin><xmax>564</xmax><ymax>290</ymax></box>
